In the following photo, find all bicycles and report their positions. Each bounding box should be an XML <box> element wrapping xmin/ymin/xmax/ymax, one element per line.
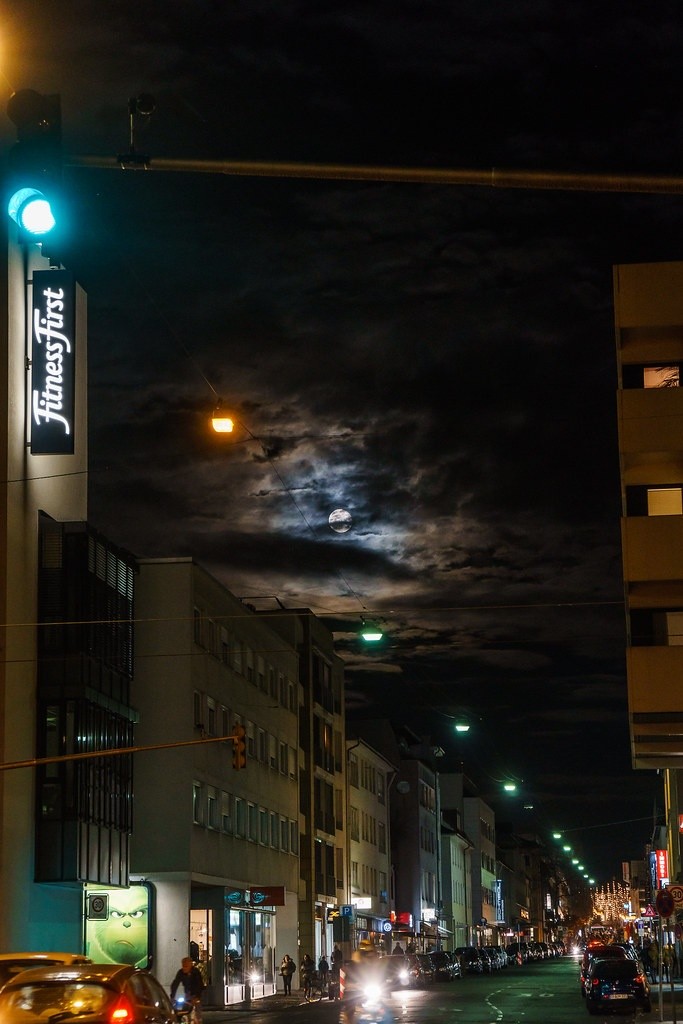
<box><xmin>304</xmin><ymin>970</ymin><xmax>337</xmax><ymax>1003</ymax></box>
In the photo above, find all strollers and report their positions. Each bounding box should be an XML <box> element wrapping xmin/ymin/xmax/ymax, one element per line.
<box><xmin>173</xmin><ymin>1000</ymin><xmax>201</xmax><ymax>1024</ymax></box>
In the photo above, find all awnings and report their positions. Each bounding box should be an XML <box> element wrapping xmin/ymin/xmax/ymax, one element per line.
<box><xmin>356</xmin><ymin>913</ymin><xmax>394</xmax><ymax>933</ymax></box>
<box><xmin>421</xmin><ymin>922</ymin><xmax>454</xmax><ymax>936</ymax></box>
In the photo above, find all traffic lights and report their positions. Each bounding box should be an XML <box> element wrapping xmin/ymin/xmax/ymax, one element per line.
<box><xmin>2</xmin><ymin>86</ymin><xmax>78</xmax><ymax>245</ymax></box>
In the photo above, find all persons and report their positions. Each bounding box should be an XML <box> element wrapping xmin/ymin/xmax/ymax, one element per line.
<box><xmin>318</xmin><ymin>955</ymin><xmax>330</xmax><ymax>988</ymax></box>
<box><xmin>171</xmin><ymin>957</ymin><xmax>203</xmax><ymax>1003</ymax></box>
<box><xmin>393</xmin><ymin>942</ymin><xmax>403</xmax><ymax>955</ymax></box>
<box><xmin>331</xmin><ymin>944</ymin><xmax>342</xmax><ymax>962</ymax></box>
<box><xmin>635</xmin><ymin>937</ymin><xmax>676</xmax><ymax>985</ymax></box>
<box><xmin>300</xmin><ymin>955</ymin><xmax>316</xmax><ymax>1000</ymax></box>
<box><xmin>279</xmin><ymin>955</ymin><xmax>296</xmax><ymax>996</ymax></box>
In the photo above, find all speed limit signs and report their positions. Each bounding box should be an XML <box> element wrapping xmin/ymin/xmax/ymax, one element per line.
<box><xmin>89</xmin><ymin>895</ymin><xmax>106</xmax><ymax>919</ymax></box>
<box><xmin>665</xmin><ymin>885</ymin><xmax>683</xmax><ymax>909</ymax></box>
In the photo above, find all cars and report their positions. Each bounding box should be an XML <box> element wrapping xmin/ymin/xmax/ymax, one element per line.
<box><xmin>376</xmin><ymin>953</ymin><xmax>436</xmax><ymax>989</ymax></box>
<box><xmin>0</xmin><ymin>952</ymin><xmax>178</xmax><ymax>1024</ymax></box>
<box><xmin>578</xmin><ymin>940</ymin><xmax>653</xmax><ymax>1013</ymax></box>
<box><xmin>503</xmin><ymin>941</ymin><xmax>573</xmax><ymax>966</ymax></box>
<box><xmin>454</xmin><ymin>945</ymin><xmax>508</xmax><ymax>975</ymax></box>
<box><xmin>343</xmin><ymin>961</ymin><xmax>392</xmax><ymax>1014</ymax></box>
<box><xmin>428</xmin><ymin>951</ymin><xmax>462</xmax><ymax>980</ymax></box>
